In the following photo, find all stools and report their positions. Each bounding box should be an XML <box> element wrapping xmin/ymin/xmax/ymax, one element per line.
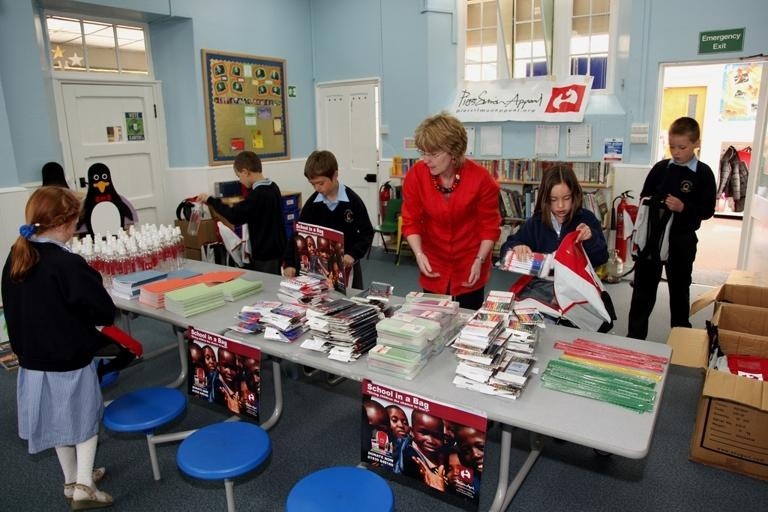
<box><xmin>176</xmin><ymin>420</ymin><xmax>272</xmax><ymax>511</ymax></box>
<box><xmin>100</xmin><ymin>370</ymin><xmax>119</xmax><ymax>389</ymax></box>
<box><xmin>285</xmin><ymin>467</ymin><xmax>394</xmax><ymax>512</ymax></box>
<box><xmin>102</xmin><ymin>387</ymin><xmax>186</xmax><ymax>481</ymax></box>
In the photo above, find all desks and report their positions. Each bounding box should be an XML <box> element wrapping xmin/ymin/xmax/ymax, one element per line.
<box><xmin>224</xmin><ymin>292</ymin><xmax>673</xmax><ymax>511</ymax></box>
<box><xmin>113</xmin><ymin>255</ymin><xmax>287</xmax><ymax>386</ymax></box>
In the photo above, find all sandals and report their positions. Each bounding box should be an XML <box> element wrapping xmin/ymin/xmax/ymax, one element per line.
<box><xmin>71</xmin><ymin>484</ymin><xmax>114</xmax><ymax>512</ymax></box>
<box><xmin>63</xmin><ymin>467</ymin><xmax>107</xmax><ymax>505</ymax></box>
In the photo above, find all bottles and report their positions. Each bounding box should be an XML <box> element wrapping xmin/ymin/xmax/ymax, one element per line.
<box><xmin>187</xmin><ymin>196</ymin><xmax>205</xmax><ymax>235</ymax></box>
<box><xmin>606</xmin><ymin>249</ymin><xmax>624</xmax><ymax>284</ymax></box>
<box><xmin>71</xmin><ymin>223</ymin><xmax>186</xmax><ymax>286</ymax></box>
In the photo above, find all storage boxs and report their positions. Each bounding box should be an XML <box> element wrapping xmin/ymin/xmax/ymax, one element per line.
<box><xmin>175</xmin><ymin>219</ymin><xmax>216</xmax><ymax>250</ymax></box>
<box><xmin>707</xmin><ymin>305</ymin><xmax>768</xmax><ymax>337</ymax></box>
<box><xmin>666</xmin><ymin>327</ymin><xmax>767</xmax><ymax>478</ymax></box>
<box><xmin>185</xmin><ymin>247</ymin><xmax>202</xmax><ymax>262</ymax></box>
<box><xmin>689</xmin><ymin>269</ymin><xmax>768</xmax><ymax>316</ymax></box>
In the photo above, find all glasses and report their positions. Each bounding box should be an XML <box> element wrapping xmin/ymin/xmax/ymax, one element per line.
<box><xmin>414</xmin><ymin>148</ymin><xmax>446</xmax><ymax>162</ymax></box>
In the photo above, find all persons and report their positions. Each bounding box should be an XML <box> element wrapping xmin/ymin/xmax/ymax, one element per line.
<box><xmin>282</xmin><ymin>150</ymin><xmax>375</xmax><ymax>291</ymax></box>
<box><xmin>2</xmin><ymin>186</ymin><xmax>119</xmax><ymax>509</ymax></box>
<box><xmin>400</xmin><ymin>110</ymin><xmax>503</xmax><ymax>311</ymax></box>
<box><xmin>291</xmin><ymin>234</ymin><xmax>346</xmax><ymax>301</ymax></box>
<box><xmin>499</xmin><ymin>162</ymin><xmax>614</xmax><ymax>334</ymax></box>
<box><xmin>358</xmin><ymin>401</ymin><xmax>485</xmax><ymax>508</ymax></box>
<box><xmin>196</xmin><ymin>150</ymin><xmax>288</xmax><ymax>276</ymax></box>
<box><xmin>625</xmin><ymin>117</ymin><xmax>717</xmax><ymax>340</ymax></box>
<box><xmin>189</xmin><ymin>341</ymin><xmax>261</xmax><ymax>423</ymax></box>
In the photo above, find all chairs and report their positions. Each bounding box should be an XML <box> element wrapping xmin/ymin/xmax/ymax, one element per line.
<box><xmin>366</xmin><ymin>198</ymin><xmax>402</xmax><ymax>261</ymax></box>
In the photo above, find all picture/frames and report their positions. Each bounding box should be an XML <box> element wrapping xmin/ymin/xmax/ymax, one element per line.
<box><xmin>201</xmin><ymin>48</ymin><xmax>292</xmax><ymax>167</ymax></box>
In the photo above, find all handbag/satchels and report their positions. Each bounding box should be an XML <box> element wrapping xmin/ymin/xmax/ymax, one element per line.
<box><xmin>737</xmin><ymin>148</ymin><xmax>751</xmax><ymax>168</ymax></box>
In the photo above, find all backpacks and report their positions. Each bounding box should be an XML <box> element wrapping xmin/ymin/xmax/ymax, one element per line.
<box><xmin>213</xmin><ymin>217</ymin><xmax>253</xmax><ymax>268</ymax></box>
<box><xmin>200</xmin><ymin>241</ymin><xmax>226</xmax><ymax>265</ymax></box>
<box><xmin>715</xmin><ymin>355</ymin><xmax>768</xmax><ymax>383</ymax></box>
<box><xmin>176</xmin><ymin>197</ymin><xmax>211</xmax><ymax>221</ymax></box>
<box><xmin>623</xmin><ymin>188</ymin><xmax>674</xmax><ymax>265</ymax></box>
<box><xmin>94</xmin><ymin>325</ymin><xmax>143</xmax><ymax>383</ymax></box>
<box><xmin>510</xmin><ymin>231</ymin><xmax>617</xmax><ymax>333</ymax></box>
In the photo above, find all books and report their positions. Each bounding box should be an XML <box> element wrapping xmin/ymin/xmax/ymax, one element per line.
<box><xmin>392</xmin><ymin>152</ymin><xmax>615</xmax><ymax>258</ymax></box>
<box><xmin>448</xmin><ymin>289</ymin><xmax>545</xmax><ymax>400</ymax></box>
<box><xmin>501</xmin><ymin>250</ymin><xmax>554</xmax><ymax>277</ymax></box>
<box><xmin>108</xmin><ymin>265</ymin><xmax>263</xmax><ymax>318</ymax></box>
<box><xmin>226</xmin><ymin>273</ymin><xmax>401</xmax><ymax>363</ymax></box>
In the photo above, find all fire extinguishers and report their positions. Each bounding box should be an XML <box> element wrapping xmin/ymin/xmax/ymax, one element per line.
<box><xmin>378</xmin><ymin>180</ymin><xmax>393</xmax><ymax>223</ymax></box>
<box><xmin>611</xmin><ymin>190</ymin><xmax>635</xmax><ymax>263</ymax></box>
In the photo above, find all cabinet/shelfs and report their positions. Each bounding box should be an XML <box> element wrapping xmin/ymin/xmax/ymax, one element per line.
<box><xmin>390</xmin><ymin>164</ymin><xmax>614</xmax><ymax>254</ymax></box>
<box><xmin>214</xmin><ymin>192</ymin><xmax>302</xmax><ymax>243</ymax></box>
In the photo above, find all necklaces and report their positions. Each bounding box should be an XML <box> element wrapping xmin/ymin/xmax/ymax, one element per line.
<box><xmin>431</xmin><ymin>161</ymin><xmax>463</xmax><ymax>193</ymax></box>
